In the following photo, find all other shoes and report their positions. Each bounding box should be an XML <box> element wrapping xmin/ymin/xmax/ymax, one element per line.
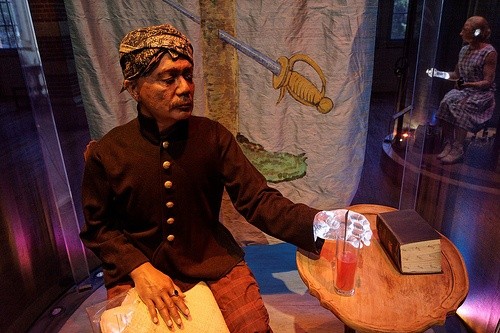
<box><xmin>436</xmin><ymin>145</ymin><xmax>465</xmax><ymax>165</ymax></box>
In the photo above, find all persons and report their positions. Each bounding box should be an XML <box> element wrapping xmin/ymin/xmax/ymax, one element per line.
<box><xmin>76</xmin><ymin>24</ymin><xmax>371</xmax><ymax>333</ymax></box>
<box><xmin>428</xmin><ymin>15</ymin><xmax>497</xmax><ymax>164</ymax></box>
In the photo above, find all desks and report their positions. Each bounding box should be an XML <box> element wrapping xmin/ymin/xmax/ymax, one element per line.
<box><xmin>296</xmin><ymin>204</ymin><xmax>470</xmax><ymax>333</ymax></box>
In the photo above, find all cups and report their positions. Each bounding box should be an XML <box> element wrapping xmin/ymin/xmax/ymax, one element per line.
<box><xmin>334</xmin><ymin>231</ymin><xmax>361</xmax><ymax>296</ymax></box>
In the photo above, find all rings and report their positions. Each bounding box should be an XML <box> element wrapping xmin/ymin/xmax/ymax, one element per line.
<box><xmin>169</xmin><ymin>290</ymin><xmax>178</xmax><ymax>296</ymax></box>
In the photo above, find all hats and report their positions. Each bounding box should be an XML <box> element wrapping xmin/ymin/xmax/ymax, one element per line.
<box><xmin>117</xmin><ymin>24</ymin><xmax>195</xmax><ymax>80</ymax></box>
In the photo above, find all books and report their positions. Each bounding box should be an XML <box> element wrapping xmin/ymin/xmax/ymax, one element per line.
<box><xmin>376</xmin><ymin>210</ymin><xmax>444</xmax><ymax>275</ymax></box>
<box><xmin>98</xmin><ymin>280</ymin><xmax>230</xmax><ymax>333</ymax></box>
